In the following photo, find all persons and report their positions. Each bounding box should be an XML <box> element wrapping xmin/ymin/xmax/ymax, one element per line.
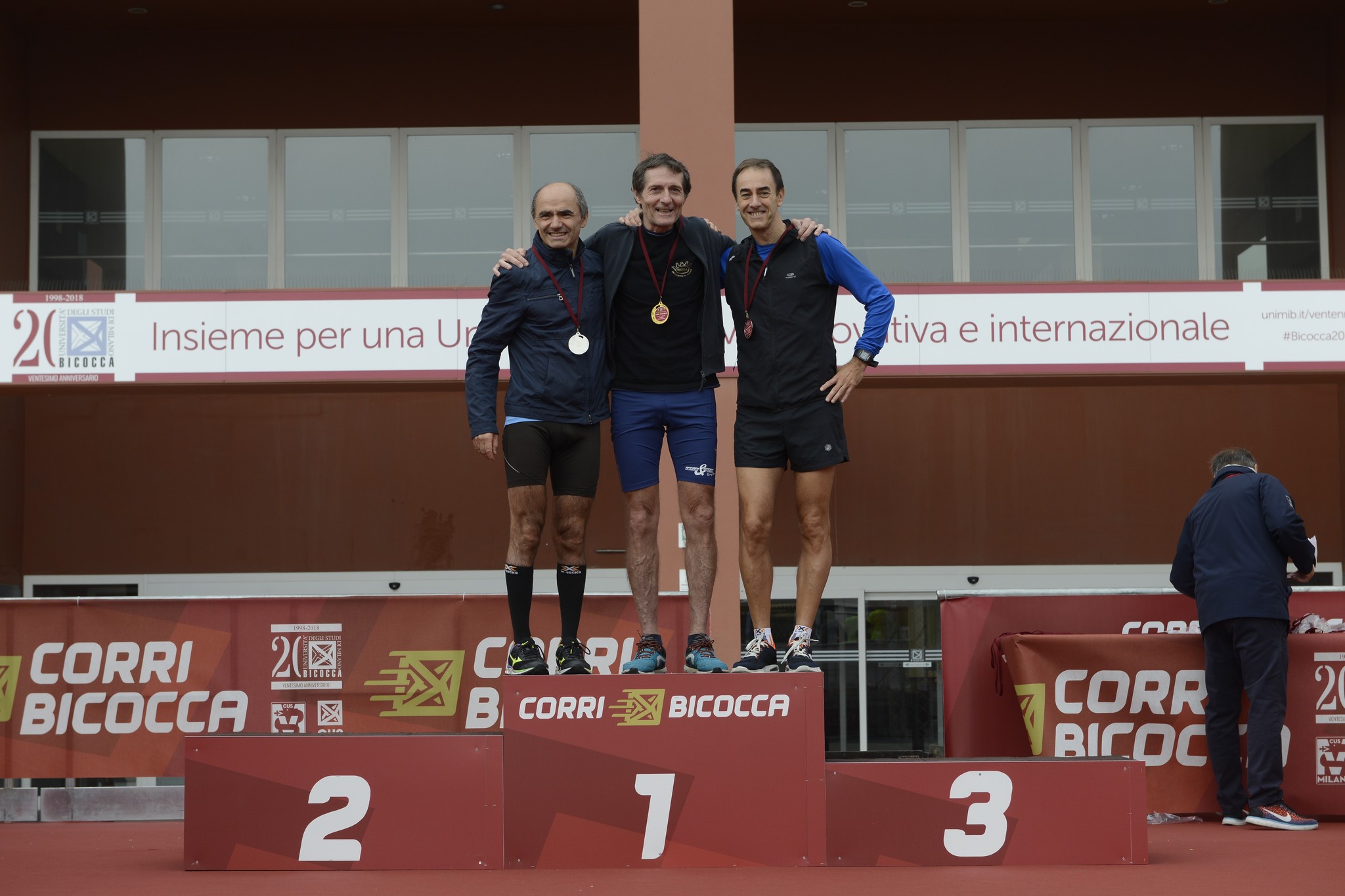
<box><xmin>711</xmin><ymin>157</ymin><xmax>896</xmax><ymax>674</ymax></box>
<box><xmin>909</xmin><ymin>703</ymin><xmax>929</xmax><ymax>752</ymax></box>
<box><xmin>1167</xmin><ymin>449</ymin><xmax>1319</xmax><ymax>830</ymax></box>
<box><xmin>464</xmin><ymin>181</ymin><xmax>605</xmax><ymax>675</ymax></box>
<box><xmin>491</xmin><ymin>150</ymin><xmax>833</xmax><ymax>674</ymax></box>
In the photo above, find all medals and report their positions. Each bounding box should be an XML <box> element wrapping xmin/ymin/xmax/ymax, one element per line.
<box><xmin>568</xmin><ymin>332</ymin><xmax>589</xmax><ymax>355</ymax></box>
<box><xmin>651</xmin><ymin>302</ymin><xmax>669</xmax><ymax>324</ymax></box>
<box><xmin>744</xmin><ymin>318</ymin><xmax>753</xmax><ymax>339</ymax></box>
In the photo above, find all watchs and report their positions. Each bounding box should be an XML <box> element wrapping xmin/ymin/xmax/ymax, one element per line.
<box><xmin>852</xmin><ymin>348</ymin><xmax>879</xmax><ymax>368</ymax></box>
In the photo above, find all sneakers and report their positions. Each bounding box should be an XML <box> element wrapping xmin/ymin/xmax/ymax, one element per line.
<box><xmin>683</xmin><ymin>633</ymin><xmax>730</xmax><ymax>673</ymax></box>
<box><xmin>780</xmin><ymin>631</ymin><xmax>822</xmax><ymax>672</ymax></box>
<box><xmin>731</xmin><ymin>629</ymin><xmax>779</xmax><ymax>673</ymax></box>
<box><xmin>505</xmin><ymin>637</ymin><xmax>549</xmax><ymax>675</ymax></box>
<box><xmin>554</xmin><ymin>638</ymin><xmax>592</xmax><ymax>674</ymax></box>
<box><xmin>1245</xmin><ymin>801</ymin><xmax>1318</xmax><ymax>830</ymax></box>
<box><xmin>1222</xmin><ymin>803</ymin><xmax>1250</xmax><ymax>825</ymax></box>
<box><xmin>621</xmin><ymin>630</ymin><xmax>667</xmax><ymax>674</ymax></box>
